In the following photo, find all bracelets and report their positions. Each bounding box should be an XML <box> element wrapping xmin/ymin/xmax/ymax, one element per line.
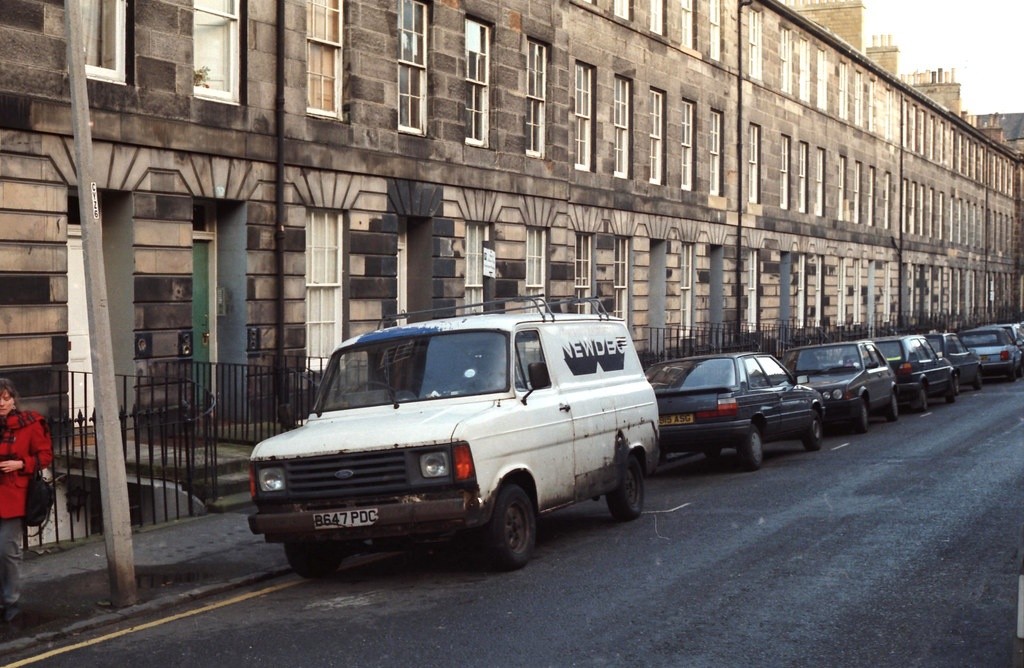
<box><xmin>21</xmin><ymin>460</ymin><xmax>25</xmax><ymax>470</ymax></box>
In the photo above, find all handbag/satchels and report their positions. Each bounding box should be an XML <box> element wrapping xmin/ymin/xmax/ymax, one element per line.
<box><xmin>23</xmin><ymin>451</ymin><xmax>53</xmax><ymax>527</ymax></box>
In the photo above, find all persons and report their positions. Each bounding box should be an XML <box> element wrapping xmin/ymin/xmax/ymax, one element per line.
<box><xmin>0</xmin><ymin>379</ymin><xmax>53</xmax><ymax>622</ymax></box>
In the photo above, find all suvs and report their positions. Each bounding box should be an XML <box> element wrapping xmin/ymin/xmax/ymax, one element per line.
<box><xmin>248</xmin><ymin>292</ymin><xmax>659</xmax><ymax>582</ymax></box>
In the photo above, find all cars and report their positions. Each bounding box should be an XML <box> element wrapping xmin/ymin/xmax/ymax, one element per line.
<box><xmin>908</xmin><ymin>331</ymin><xmax>983</xmax><ymax>397</ymax></box>
<box><xmin>769</xmin><ymin>340</ymin><xmax>901</xmax><ymax>436</ymax></box>
<box><xmin>948</xmin><ymin>321</ymin><xmax>1024</xmax><ymax>385</ymax></box>
<box><xmin>638</xmin><ymin>351</ymin><xmax>826</xmax><ymax>473</ymax></box>
<box><xmin>829</xmin><ymin>334</ymin><xmax>956</xmax><ymax>413</ymax></box>
